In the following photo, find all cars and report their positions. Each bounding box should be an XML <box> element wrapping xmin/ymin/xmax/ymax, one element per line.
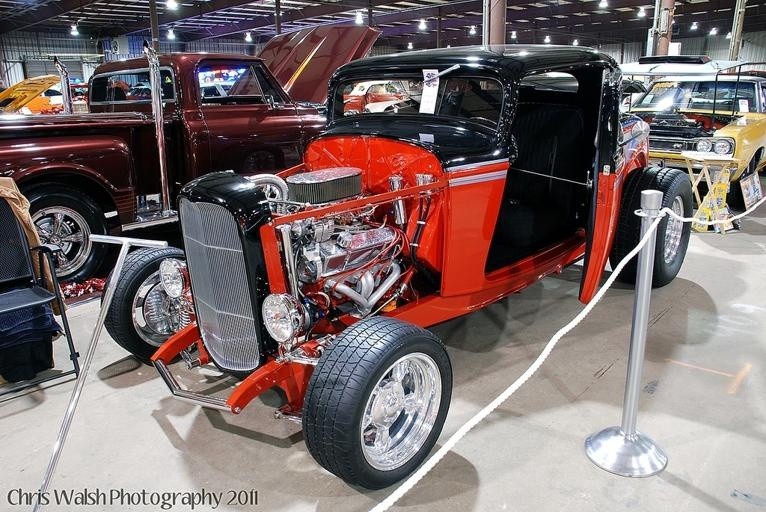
<box><xmin>98</xmin><ymin>42</ymin><xmax>693</xmax><ymax>489</ymax></box>
<box><xmin>21</xmin><ymin>88</ymin><xmax>64</xmax><ymax>116</ymax></box>
<box><xmin>611</xmin><ymin>53</ymin><xmax>766</xmax><ymax>213</ymax></box>
<box><xmin>344</xmin><ymin>79</ymin><xmax>422</xmax><ymax>115</ymax></box>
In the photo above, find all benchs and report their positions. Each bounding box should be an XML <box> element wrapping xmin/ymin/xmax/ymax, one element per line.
<box><xmin>497</xmin><ymin>99</ymin><xmax>578</xmax><ymax>246</ymax></box>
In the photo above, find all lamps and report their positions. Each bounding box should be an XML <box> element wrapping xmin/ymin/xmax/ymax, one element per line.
<box><xmin>245</xmin><ymin>32</ymin><xmax>252</xmax><ymax>42</ymax></box>
<box><xmin>70</xmin><ymin>23</ymin><xmax>79</xmax><ymax>37</ymax></box>
<box><xmin>166</xmin><ymin>28</ymin><xmax>176</xmax><ymax>40</ymax></box>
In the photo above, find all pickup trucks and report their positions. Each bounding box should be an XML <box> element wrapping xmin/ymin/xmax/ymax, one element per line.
<box><xmin>1</xmin><ymin>25</ymin><xmax>386</xmax><ymax>293</ymax></box>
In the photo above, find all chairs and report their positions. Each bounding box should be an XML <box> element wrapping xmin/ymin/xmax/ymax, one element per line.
<box><xmin>107</xmin><ymin>88</ymin><xmax>127</xmax><ymax>102</ymax></box>
<box><xmin>161</xmin><ymin>83</ymin><xmax>174</xmax><ymax>98</ymax></box>
<box><xmin>0</xmin><ymin>177</ymin><xmax>80</xmax><ymax>399</ymax></box>
